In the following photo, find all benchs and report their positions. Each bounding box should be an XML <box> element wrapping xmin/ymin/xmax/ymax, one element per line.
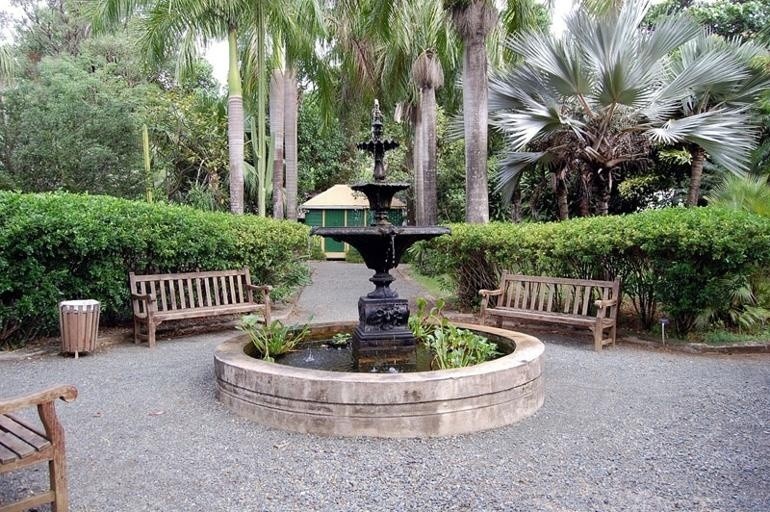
<box><xmin>478</xmin><ymin>268</ymin><xmax>620</xmax><ymax>353</ymax></box>
<box><xmin>0</xmin><ymin>382</ymin><xmax>78</xmax><ymax>512</ymax></box>
<box><xmin>128</xmin><ymin>266</ymin><xmax>274</xmax><ymax>348</ymax></box>
